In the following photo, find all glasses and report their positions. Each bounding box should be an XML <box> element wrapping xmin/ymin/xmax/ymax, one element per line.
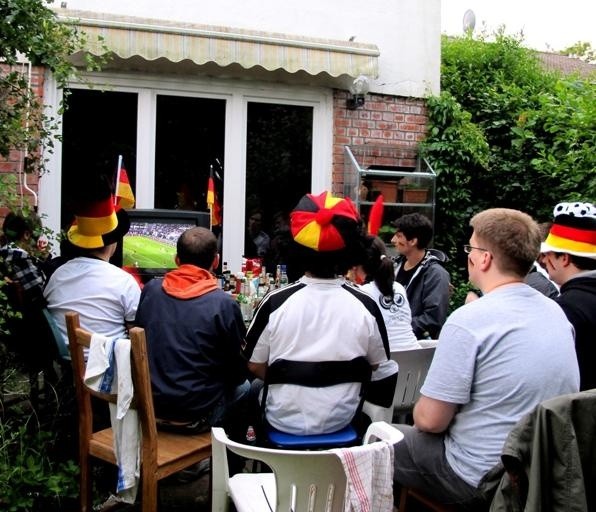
<box><xmin>463</xmin><ymin>245</ymin><xmax>493</xmax><ymax>260</ymax></box>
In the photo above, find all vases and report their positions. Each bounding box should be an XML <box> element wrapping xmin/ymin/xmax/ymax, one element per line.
<box><xmin>352</xmin><ymin>179</ymin><xmax>398</xmax><ymax>202</ymax></box>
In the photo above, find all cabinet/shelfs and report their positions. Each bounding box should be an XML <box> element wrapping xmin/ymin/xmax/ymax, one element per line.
<box><xmin>343</xmin><ymin>144</ymin><xmax>436</xmax><ymax>256</ymax></box>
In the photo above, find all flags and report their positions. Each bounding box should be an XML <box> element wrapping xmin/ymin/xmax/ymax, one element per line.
<box><xmin>204</xmin><ymin>164</ymin><xmax>224</xmax><ymax>226</ymax></box>
<box><xmin>112</xmin><ymin>153</ymin><xmax>135</xmax><ymax>209</ymax></box>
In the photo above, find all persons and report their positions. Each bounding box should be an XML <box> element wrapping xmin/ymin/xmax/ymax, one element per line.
<box><xmin>43</xmin><ymin>191</ymin><xmax>142</xmax><ymax>363</ymax></box>
<box><xmin>341</xmin><ymin>213</ymin><xmax>453</xmax><ymax>345</ymax></box>
<box><xmin>239</xmin><ymin>191</ymin><xmax>390</xmax><ymax>435</ymax></box>
<box><xmin>376</xmin><ymin>208</ymin><xmax>580</xmax><ymax>511</ymax></box>
<box><xmin>465</xmin><ymin>202</ymin><xmax>596</xmax><ymax>392</ymax></box>
<box><xmin>245</xmin><ymin>212</ymin><xmax>304</xmax><ymax>284</ymax></box>
<box><xmin>1</xmin><ymin>209</ymin><xmax>67</xmax><ymax>368</ymax></box>
<box><xmin>123</xmin><ymin>222</ymin><xmax>196</xmax><ymax>269</ymax></box>
<box><xmin>131</xmin><ymin>226</ymin><xmax>253</xmax><ymax>511</ymax></box>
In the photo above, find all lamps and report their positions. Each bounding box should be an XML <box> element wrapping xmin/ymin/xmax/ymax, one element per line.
<box><xmin>346</xmin><ymin>75</ymin><xmax>369</xmax><ymax>110</ymax></box>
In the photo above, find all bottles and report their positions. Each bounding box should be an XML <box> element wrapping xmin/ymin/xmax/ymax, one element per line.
<box><xmin>222</xmin><ymin>262</ymin><xmax>288</xmax><ymax>327</ymax></box>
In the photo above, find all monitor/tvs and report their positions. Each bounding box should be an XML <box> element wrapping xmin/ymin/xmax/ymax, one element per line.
<box><xmin>113</xmin><ymin>210</ymin><xmax>210</xmax><ymax>282</ymax></box>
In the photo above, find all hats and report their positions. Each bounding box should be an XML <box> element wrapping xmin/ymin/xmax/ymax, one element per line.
<box><xmin>290</xmin><ymin>190</ymin><xmax>359</xmax><ymax>252</ymax></box>
<box><xmin>540</xmin><ymin>214</ymin><xmax>596</xmax><ymax>260</ymax></box>
<box><xmin>66</xmin><ymin>188</ymin><xmax>130</xmax><ymax>249</ymax></box>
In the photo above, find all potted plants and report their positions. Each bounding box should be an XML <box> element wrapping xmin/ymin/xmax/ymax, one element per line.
<box><xmin>402</xmin><ymin>182</ymin><xmax>428</xmax><ymax>202</ymax></box>
